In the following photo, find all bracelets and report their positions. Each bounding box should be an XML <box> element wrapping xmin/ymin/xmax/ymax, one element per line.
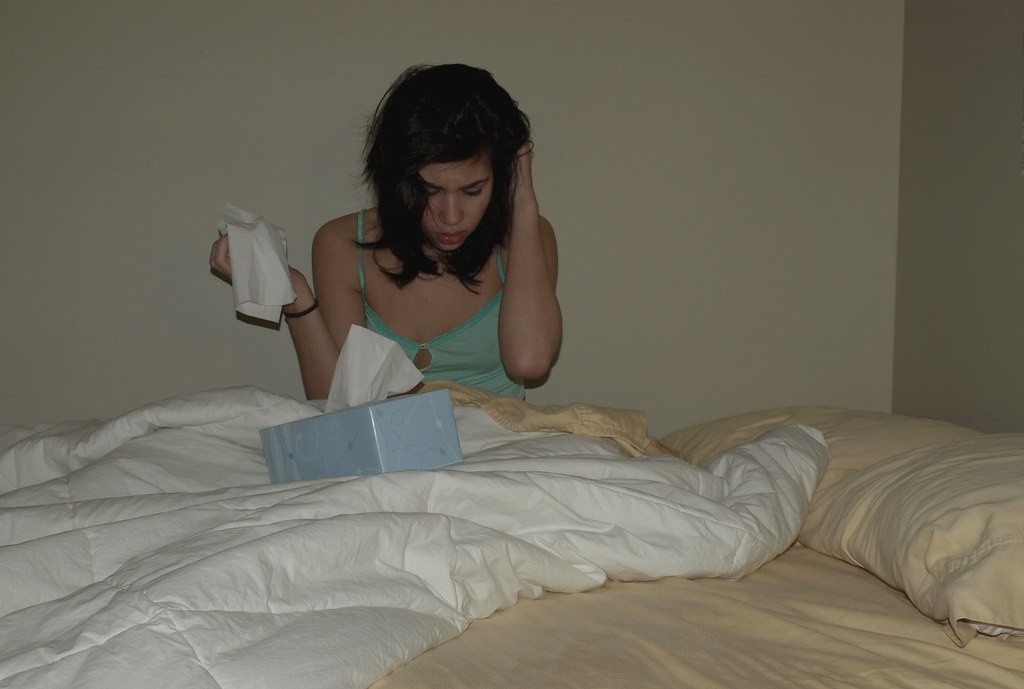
<box><xmin>282</xmin><ymin>298</ymin><xmax>319</xmax><ymax>318</ymax></box>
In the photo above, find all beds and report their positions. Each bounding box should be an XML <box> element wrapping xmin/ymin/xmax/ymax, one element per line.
<box><xmin>1</xmin><ymin>381</ymin><xmax>1024</xmax><ymax>689</ymax></box>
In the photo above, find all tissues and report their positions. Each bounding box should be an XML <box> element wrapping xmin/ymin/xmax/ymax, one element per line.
<box><xmin>257</xmin><ymin>323</ymin><xmax>465</xmax><ymax>485</ymax></box>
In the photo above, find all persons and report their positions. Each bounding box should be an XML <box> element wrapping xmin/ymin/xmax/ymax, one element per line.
<box><xmin>209</xmin><ymin>64</ymin><xmax>562</xmax><ymax>400</ymax></box>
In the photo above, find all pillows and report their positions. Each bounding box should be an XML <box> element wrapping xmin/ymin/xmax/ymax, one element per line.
<box><xmin>659</xmin><ymin>405</ymin><xmax>981</xmax><ymax>550</ymax></box>
<box><xmin>794</xmin><ymin>431</ymin><xmax>1024</xmax><ymax>648</ymax></box>
